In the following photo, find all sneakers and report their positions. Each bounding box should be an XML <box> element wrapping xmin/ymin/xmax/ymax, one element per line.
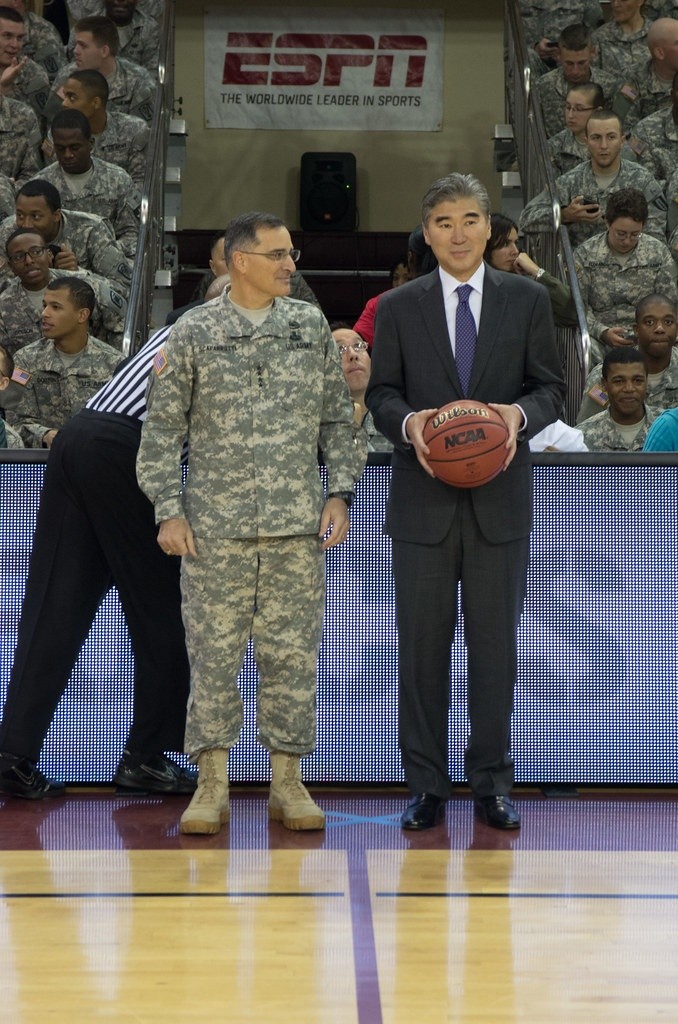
<box><xmin>0</xmin><ymin>757</ymin><xmax>65</xmax><ymax>798</ymax></box>
<box><xmin>112</xmin><ymin>748</ymin><xmax>199</xmax><ymax>792</ymax></box>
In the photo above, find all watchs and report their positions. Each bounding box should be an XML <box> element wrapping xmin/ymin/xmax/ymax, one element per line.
<box><xmin>329</xmin><ymin>490</ymin><xmax>355</xmax><ymax>506</ymax></box>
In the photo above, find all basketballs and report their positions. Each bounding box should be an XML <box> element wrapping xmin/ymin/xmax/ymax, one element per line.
<box><xmin>421</xmin><ymin>399</ymin><xmax>510</xmax><ymax>490</ymax></box>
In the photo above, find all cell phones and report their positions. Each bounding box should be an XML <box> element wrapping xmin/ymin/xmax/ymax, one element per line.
<box><xmin>583</xmin><ymin>197</ymin><xmax>599</xmax><ymax>213</ymax></box>
<box><xmin>546</xmin><ymin>42</ymin><xmax>559</xmax><ymax>48</ymax></box>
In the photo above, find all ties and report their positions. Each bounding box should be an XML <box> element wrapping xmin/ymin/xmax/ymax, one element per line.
<box><xmin>454</xmin><ymin>284</ymin><xmax>478</xmax><ymax>398</ymax></box>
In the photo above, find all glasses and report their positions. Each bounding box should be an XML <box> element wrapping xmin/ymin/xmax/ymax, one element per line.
<box><xmin>565</xmin><ymin>105</ymin><xmax>598</xmax><ymax>111</ymax></box>
<box><xmin>338</xmin><ymin>341</ymin><xmax>369</xmax><ymax>354</ymax></box>
<box><xmin>610</xmin><ymin>225</ymin><xmax>643</xmax><ymax>239</ymax></box>
<box><xmin>234</xmin><ymin>249</ymin><xmax>301</xmax><ymax>262</ymax></box>
<box><xmin>9</xmin><ymin>246</ymin><xmax>49</xmax><ymax>264</ymax></box>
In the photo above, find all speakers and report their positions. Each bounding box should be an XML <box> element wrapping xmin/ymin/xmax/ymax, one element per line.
<box><xmin>300</xmin><ymin>152</ymin><xmax>356</xmax><ymax>230</ymax></box>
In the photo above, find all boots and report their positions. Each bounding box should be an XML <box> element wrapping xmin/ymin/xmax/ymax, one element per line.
<box><xmin>268</xmin><ymin>751</ymin><xmax>326</xmax><ymax>830</ymax></box>
<box><xmin>179</xmin><ymin>749</ymin><xmax>230</xmax><ymax>833</ymax></box>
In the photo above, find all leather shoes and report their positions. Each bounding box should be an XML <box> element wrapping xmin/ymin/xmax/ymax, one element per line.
<box><xmin>473</xmin><ymin>790</ymin><xmax>521</xmax><ymax>829</ymax></box>
<box><xmin>401</xmin><ymin>793</ymin><xmax>445</xmax><ymax>829</ymax></box>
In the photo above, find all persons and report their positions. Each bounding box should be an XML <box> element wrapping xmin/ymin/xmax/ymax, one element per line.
<box><xmin>0</xmin><ymin>0</ymin><xmax>167</xmax><ymax>451</ymax></box>
<box><xmin>0</xmin><ymin>273</ymin><xmax>244</xmax><ymax>799</ymax></box>
<box><xmin>134</xmin><ymin>209</ymin><xmax>369</xmax><ymax>835</ymax></box>
<box><xmin>363</xmin><ymin>171</ymin><xmax>567</xmax><ymax>831</ymax></box>
<box><xmin>507</xmin><ymin>0</ymin><xmax>678</xmax><ymax>453</ymax></box>
<box><xmin>170</xmin><ymin>211</ymin><xmax>579</xmax><ymax>450</ymax></box>
